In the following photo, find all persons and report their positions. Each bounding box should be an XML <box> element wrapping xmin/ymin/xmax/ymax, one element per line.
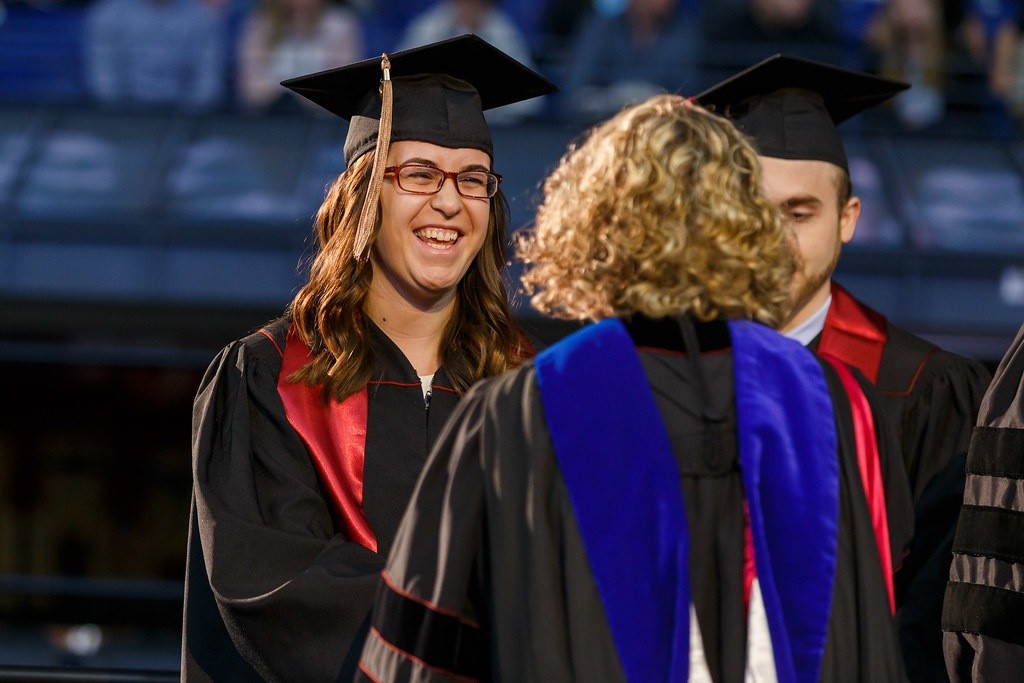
<box><xmin>75</xmin><ymin>2</ymin><xmax>1019</xmax><ymax>126</ymax></box>
<box><xmin>162</xmin><ymin>28</ymin><xmax>530</xmax><ymax>681</ymax></box>
<box><xmin>357</xmin><ymin>100</ymin><xmax>909</xmax><ymax>683</ymax></box>
<box><xmin>687</xmin><ymin>47</ymin><xmax>982</xmax><ymax>683</ymax></box>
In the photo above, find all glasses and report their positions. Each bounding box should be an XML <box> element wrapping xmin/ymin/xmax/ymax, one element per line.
<box><xmin>378</xmin><ymin>163</ymin><xmax>504</xmax><ymax>199</ymax></box>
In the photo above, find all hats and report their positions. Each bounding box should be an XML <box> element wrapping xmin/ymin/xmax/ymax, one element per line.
<box><xmin>687</xmin><ymin>52</ymin><xmax>913</xmax><ymax>170</ymax></box>
<box><xmin>280</xmin><ymin>35</ymin><xmax>561</xmax><ymax>262</ymax></box>
